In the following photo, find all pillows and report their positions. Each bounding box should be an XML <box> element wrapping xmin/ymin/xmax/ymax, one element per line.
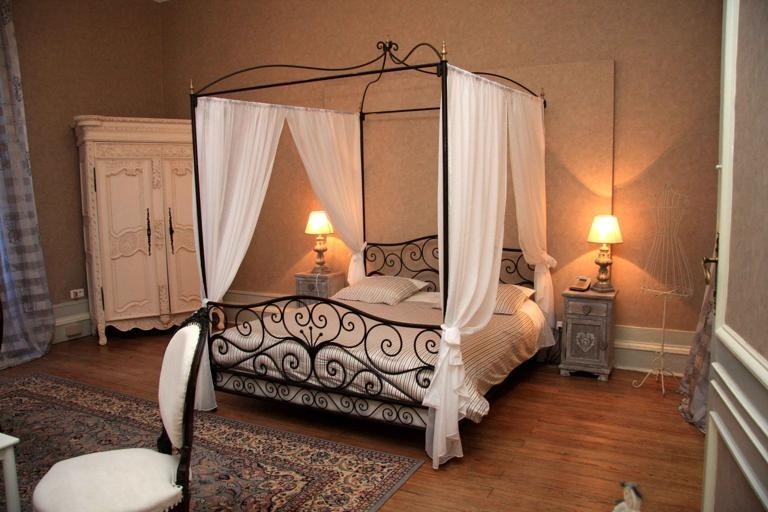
<box><xmin>336</xmin><ymin>276</ymin><xmax>429</xmax><ymax>306</ymax></box>
<box><xmin>432</xmin><ymin>283</ymin><xmax>536</xmax><ymax>315</ymax></box>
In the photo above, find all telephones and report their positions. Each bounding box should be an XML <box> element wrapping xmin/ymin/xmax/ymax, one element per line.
<box><xmin>569</xmin><ymin>276</ymin><xmax>591</xmax><ymax>291</ymax></box>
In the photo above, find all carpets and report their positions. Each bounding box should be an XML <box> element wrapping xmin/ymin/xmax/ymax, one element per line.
<box><xmin>0</xmin><ymin>370</ymin><xmax>427</xmax><ymax>512</ymax></box>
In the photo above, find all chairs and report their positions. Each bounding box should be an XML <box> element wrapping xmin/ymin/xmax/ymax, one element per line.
<box><xmin>32</xmin><ymin>314</ymin><xmax>207</xmax><ymax>512</ymax></box>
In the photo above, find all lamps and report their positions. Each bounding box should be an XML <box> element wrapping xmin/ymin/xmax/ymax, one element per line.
<box><xmin>304</xmin><ymin>211</ymin><xmax>334</xmax><ymax>273</ymax></box>
<box><xmin>587</xmin><ymin>215</ymin><xmax>623</xmax><ymax>293</ymax></box>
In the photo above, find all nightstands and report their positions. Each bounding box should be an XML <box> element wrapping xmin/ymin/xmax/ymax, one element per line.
<box><xmin>294</xmin><ymin>272</ymin><xmax>345</xmax><ymax>308</ymax></box>
<box><xmin>559</xmin><ymin>288</ymin><xmax>619</xmax><ymax>382</ymax></box>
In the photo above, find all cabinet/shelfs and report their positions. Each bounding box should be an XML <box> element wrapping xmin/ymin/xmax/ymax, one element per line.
<box><xmin>69</xmin><ymin>115</ymin><xmax>206</xmax><ymax>346</ymax></box>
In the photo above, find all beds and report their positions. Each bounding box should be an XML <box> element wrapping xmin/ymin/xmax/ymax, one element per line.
<box><xmin>187</xmin><ymin>40</ymin><xmax>557</xmax><ymax>471</ymax></box>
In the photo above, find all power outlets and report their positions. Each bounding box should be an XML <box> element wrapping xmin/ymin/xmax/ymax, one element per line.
<box><xmin>70</xmin><ymin>288</ymin><xmax>84</xmax><ymax>300</ymax></box>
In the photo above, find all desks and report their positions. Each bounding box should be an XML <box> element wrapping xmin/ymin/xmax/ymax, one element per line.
<box><xmin>0</xmin><ymin>432</ymin><xmax>21</xmax><ymax>512</ymax></box>
